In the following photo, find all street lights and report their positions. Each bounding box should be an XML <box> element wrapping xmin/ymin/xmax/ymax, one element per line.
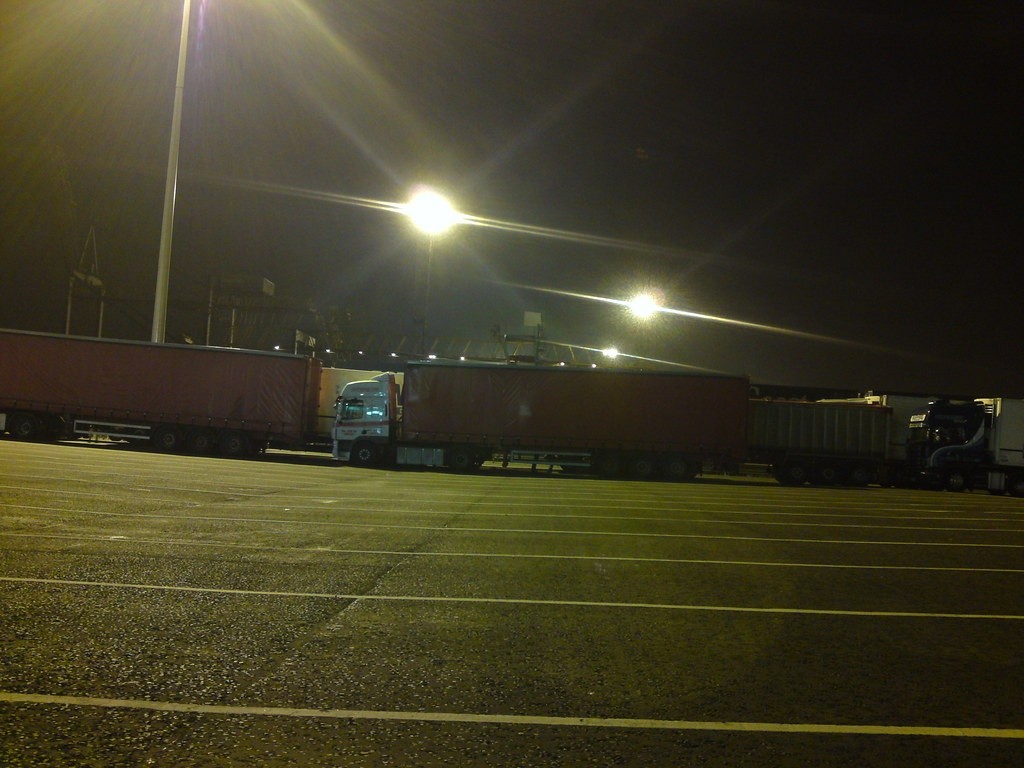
<box><xmin>406</xmin><ymin>186</ymin><xmax>454</xmax><ymax>357</ymax></box>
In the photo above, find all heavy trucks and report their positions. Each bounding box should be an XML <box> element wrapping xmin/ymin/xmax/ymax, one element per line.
<box><xmin>331</xmin><ymin>357</ymin><xmax>749</xmax><ymax>483</ymax></box>
<box><xmin>904</xmin><ymin>396</ymin><xmax>1024</xmax><ymax>499</ymax></box>
